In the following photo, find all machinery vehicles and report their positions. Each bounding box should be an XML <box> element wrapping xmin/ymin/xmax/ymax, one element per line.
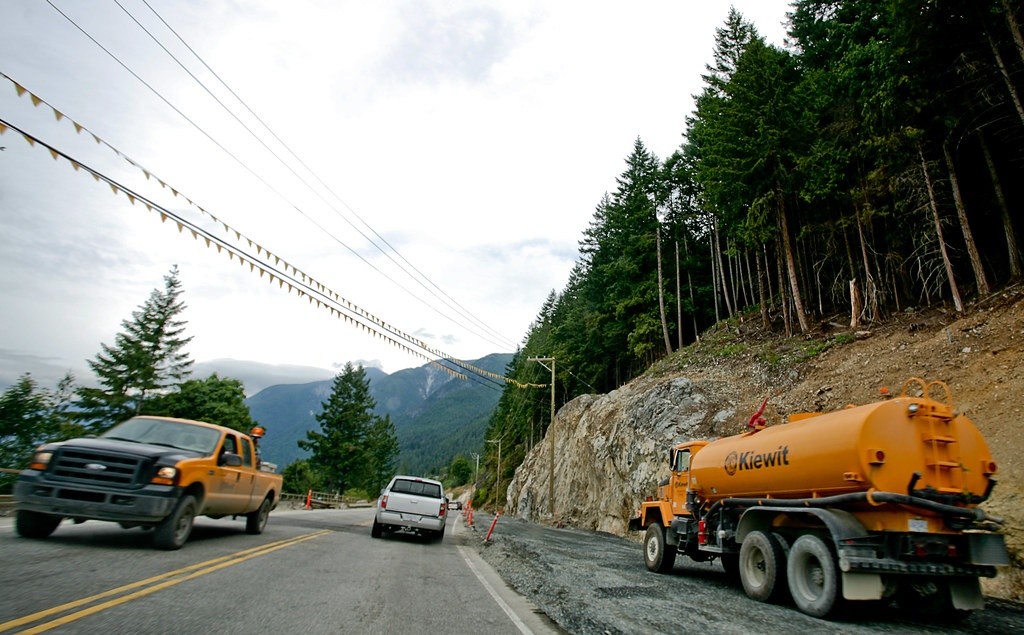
<box><xmin>640</xmin><ymin>376</ymin><xmax>1012</xmax><ymax>619</ymax></box>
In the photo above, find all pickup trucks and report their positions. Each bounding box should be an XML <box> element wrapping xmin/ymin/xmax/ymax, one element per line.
<box><xmin>15</xmin><ymin>416</ymin><xmax>285</xmax><ymax>552</ymax></box>
<box><xmin>372</xmin><ymin>475</ymin><xmax>450</xmax><ymax>543</ymax></box>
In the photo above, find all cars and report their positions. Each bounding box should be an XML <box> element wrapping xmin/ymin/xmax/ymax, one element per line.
<box><xmin>448</xmin><ymin>501</ymin><xmax>462</xmax><ymax>510</ymax></box>
